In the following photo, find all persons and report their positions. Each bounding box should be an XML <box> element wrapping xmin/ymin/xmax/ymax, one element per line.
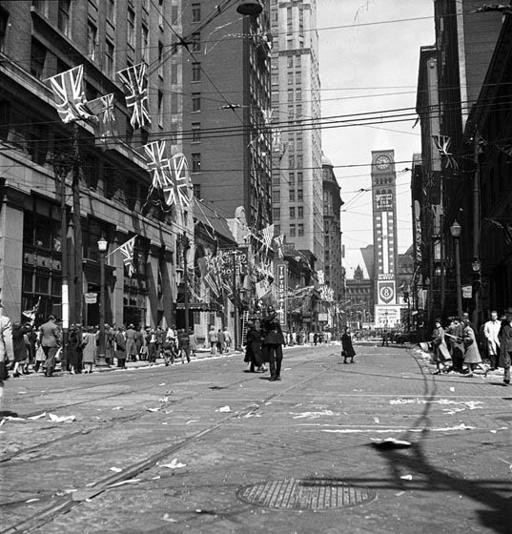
<box><xmin>1</xmin><ymin>306</ymin><xmax>191</xmax><ymax>382</ymax></box>
<box><xmin>208</xmin><ymin>327</ymin><xmax>232</xmax><ymax>355</ymax></box>
<box><xmin>289</xmin><ymin>328</ymin><xmax>324</xmax><ymax>345</ymax></box>
<box><xmin>245</xmin><ymin>305</ymin><xmax>287</xmax><ymax>382</ymax></box>
<box><xmin>431</xmin><ymin>307</ymin><xmax>512</xmax><ymax>385</ymax></box>
<box><xmin>340</xmin><ymin>327</ymin><xmax>357</xmax><ymax>364</ymax></box>
<box><xmin>381</xmin><ymin>327</ymin><xmax>390</xmax><ymax>348</ymax></box>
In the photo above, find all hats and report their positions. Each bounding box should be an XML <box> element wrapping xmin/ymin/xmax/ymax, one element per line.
<box><xmin>435</xmin><ymin>317</ymin><xmax>443</xmax><ymax>323</ymax></box>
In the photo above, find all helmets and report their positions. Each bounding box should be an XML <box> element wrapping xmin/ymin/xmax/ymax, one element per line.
<box><xmin>267</xmin><ymin>305</ymin><xmax>275</xmax><ymax>312</ymax></box>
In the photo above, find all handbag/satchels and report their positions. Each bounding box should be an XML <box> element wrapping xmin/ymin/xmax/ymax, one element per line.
<box><xmin>341</xmin><ymin>351</ymin><xmax>349</xmax><ymax>357</ymax></box>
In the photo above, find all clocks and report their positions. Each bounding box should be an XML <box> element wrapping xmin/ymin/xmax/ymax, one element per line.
<box><xmin>376</xmin><ymin>154</ymin><xmax>392</xmax><ymax>170</ymax></box>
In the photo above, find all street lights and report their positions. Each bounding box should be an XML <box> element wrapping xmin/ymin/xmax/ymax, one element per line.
<box><xmin>448</xmin><ymin>218</ymin><xmax>463</xmax><ymax>319</ymax></box>
<box><xmin>96</xmin><ymin>235</ymin><xmax>110</xmax><ymax>369</ymax></box>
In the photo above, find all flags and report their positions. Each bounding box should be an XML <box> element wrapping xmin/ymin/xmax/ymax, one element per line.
<box><xmin>433</xmin><ymin>133</ymin><xmax>459</xmax><ymax>170</ymax></box>
<box><xmin>142</xmin><ymin>137</ymin><xmax>194</xmax><ymax>209</ymax></box>
<box><xmin>117</xmin><ymin>239</ymin><xmax>136</xmax><ymax>280</ymax></box>
<box><xmin>260</xmin><ymin>224</ymin><xmax>284</xmax><ymax>259</ymax></box>
<box><xmin>49</xmin><ymin>64</ymin><xmax>151</xmax><ymax>148</ymax></box>
